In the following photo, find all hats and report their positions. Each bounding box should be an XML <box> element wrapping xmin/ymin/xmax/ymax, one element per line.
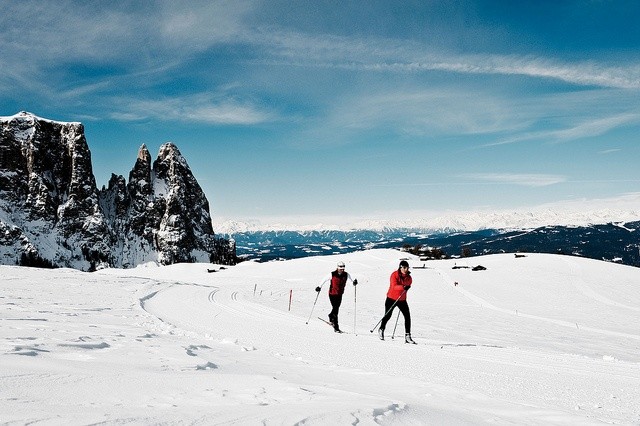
<box><xmin>399</xmin><ymin>261</ymin><xmax>409</xmax><ymax>269</ymax></box>
<box><xmin>337</xmin><ymin>261</ymin><xmax>345</xmax><ymax>269</ymax></box>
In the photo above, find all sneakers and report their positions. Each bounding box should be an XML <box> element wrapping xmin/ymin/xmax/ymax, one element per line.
<box><xmin>405</xmin><ymin>333</ymin><xmax>415</xmax><ymax>344</ymax></box>
<box><xmin>378</xmin><ymin>328</ymin><xmax>384</xmax><ymax>340</ymax></box>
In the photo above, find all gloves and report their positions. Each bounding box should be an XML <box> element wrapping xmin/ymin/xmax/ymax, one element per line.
<box><xmin>316</xmin><ymin>287</ymin><xmax>321</xmax><ymax>291</ymax></box>
<box><xmin>405</xmin><ymin>285</ymin><xmax>410</xmax><ymax>290</ymax></box>
<box><xmin>353</xmin><ymin>280</ymin><xmax>357</xmax><ymax>285</ymax></box>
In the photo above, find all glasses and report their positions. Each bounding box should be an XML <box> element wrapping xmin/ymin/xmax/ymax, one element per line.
<box><xmin>339</xmin><ymin>265</ymin><xmax>345</xmax><ymax>267</ymax></box>
<box><xmin>399</xmin><ymin>265</ymin><xmax>409</xmax><ymax>272</ymax></box>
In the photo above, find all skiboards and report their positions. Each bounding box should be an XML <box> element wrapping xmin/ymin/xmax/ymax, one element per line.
<box><xmin>317</xmin><ymin>316</ymin><xmax>352</xmax><ymax>333</ymax></box>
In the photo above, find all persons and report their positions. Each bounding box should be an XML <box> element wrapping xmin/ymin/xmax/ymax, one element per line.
<box><xmin>378</xmin><ymin>261</ymin><xmax>415</xmax><ymax>344</ymax></box>
<box><xmin>315</xmin><ymin>262</ymin><xmax>358</xmax><ymax>334</ymax></box>
